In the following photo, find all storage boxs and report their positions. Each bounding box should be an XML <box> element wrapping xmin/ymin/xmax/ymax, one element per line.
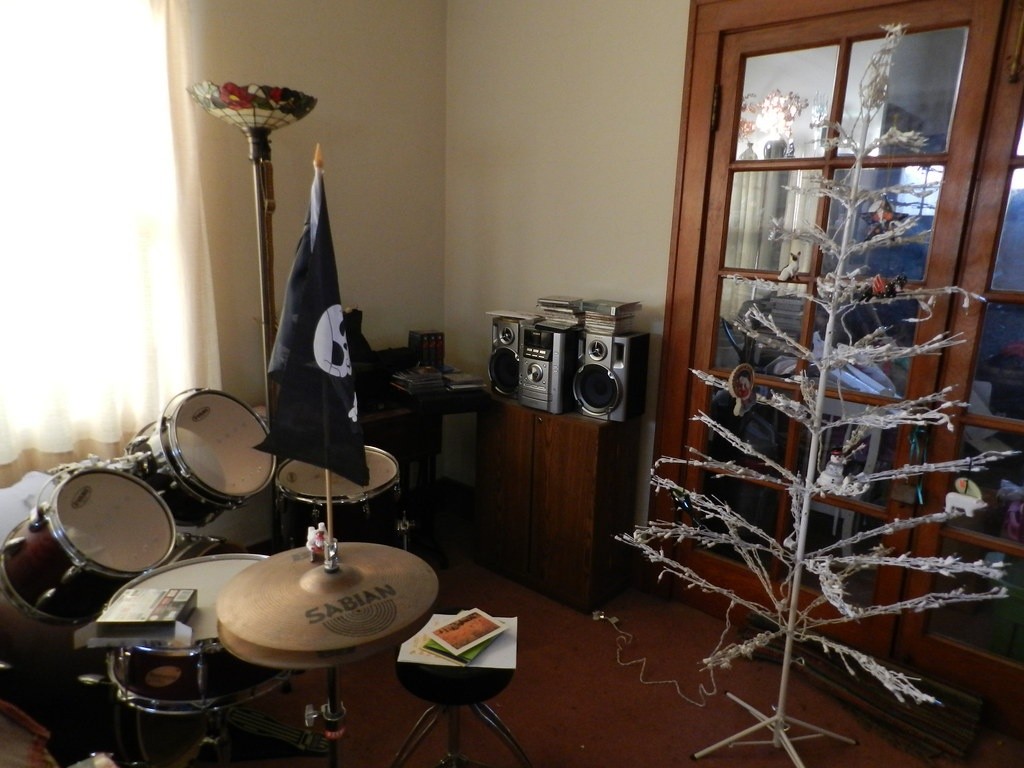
<box><xmin>882</xmin><ymin>365</ymin><xmax>1016</xmax><ymax>616</ymax></box>
<box><xmin>75</xmin><ymin>589</ymin><xmax>197</xmax><ymax>649</ymax></box>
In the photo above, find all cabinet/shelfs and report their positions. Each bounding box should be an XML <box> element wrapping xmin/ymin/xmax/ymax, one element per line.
<box><xmin>471</xmin><ymin>381</ymin><xmax>642</xmax><ymax>615</ymax></box>
<box><xmin>297</xmin><ymin>381</ymin><xmax>478</xmax><ymax>544</ymax></box>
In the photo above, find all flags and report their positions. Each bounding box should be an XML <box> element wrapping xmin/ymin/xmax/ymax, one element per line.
<box><xmin>253</xmin><ymin>169</ymin><xmax>370</xmax><ymax>487</ymax></box>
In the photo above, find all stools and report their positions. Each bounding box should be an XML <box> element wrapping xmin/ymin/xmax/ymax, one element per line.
<box><xmin>389</xmin><ymin>641</ymin><xmax>533</xmax><ymax>768</ymax></box>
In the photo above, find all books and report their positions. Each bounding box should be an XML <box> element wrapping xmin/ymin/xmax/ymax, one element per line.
<box><xmin>756</xmin><ymin>294</ymin><xmax>806</xmax><ymax>341</ymax></box>
<box><xmin>534</xmin><ymin>296</ymin><xmax>643</xmax><ymax>336</ymax></box>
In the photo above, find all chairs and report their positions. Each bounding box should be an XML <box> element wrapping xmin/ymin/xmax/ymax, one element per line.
<box><xmin>794</xmin><ymin>397</ymin><xmax>883</xmax><ymax>567</ymax></box>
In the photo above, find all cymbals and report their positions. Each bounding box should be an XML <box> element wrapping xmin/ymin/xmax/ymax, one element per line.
<box><xmin>215</xmin><ymin>539</ymin><xmax>440</xmax><ymax>670</ymax></box>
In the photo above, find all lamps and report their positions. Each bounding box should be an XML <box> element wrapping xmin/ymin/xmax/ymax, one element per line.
<box><xmin>184</xmin><ymin>80</ymin><xmax>322</xmax><ymax>556</ymax></box>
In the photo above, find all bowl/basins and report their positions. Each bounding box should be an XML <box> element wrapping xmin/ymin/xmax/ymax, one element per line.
<box><xmin>306</xmin><ymin>538</ymin><xmax>338</xmax><ymax>563</ymax></box>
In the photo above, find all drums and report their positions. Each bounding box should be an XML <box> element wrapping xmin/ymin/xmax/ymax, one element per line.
<box><xmin>124</xmin><ymin>386</ymin><xmax>278</xmax><ymax>526</ymax></box>
<box><xmin>1</xmin><ymin>642</ymin><xmax>209</xmax><ymax>768</ymax></box>
<box><xmin>0</xmin><ymin>462</ymin><xmax>178</xmax><ymax>627</ymax></box>
<box><xmin>273</xmin><ymin>443</ymin><xmax>403</xmax><ymax>551</ymax></box>
<box><xmin>100</xmin><ymin>550</ymin><xmax>292</xmax><ymax>716</ymax></box>
